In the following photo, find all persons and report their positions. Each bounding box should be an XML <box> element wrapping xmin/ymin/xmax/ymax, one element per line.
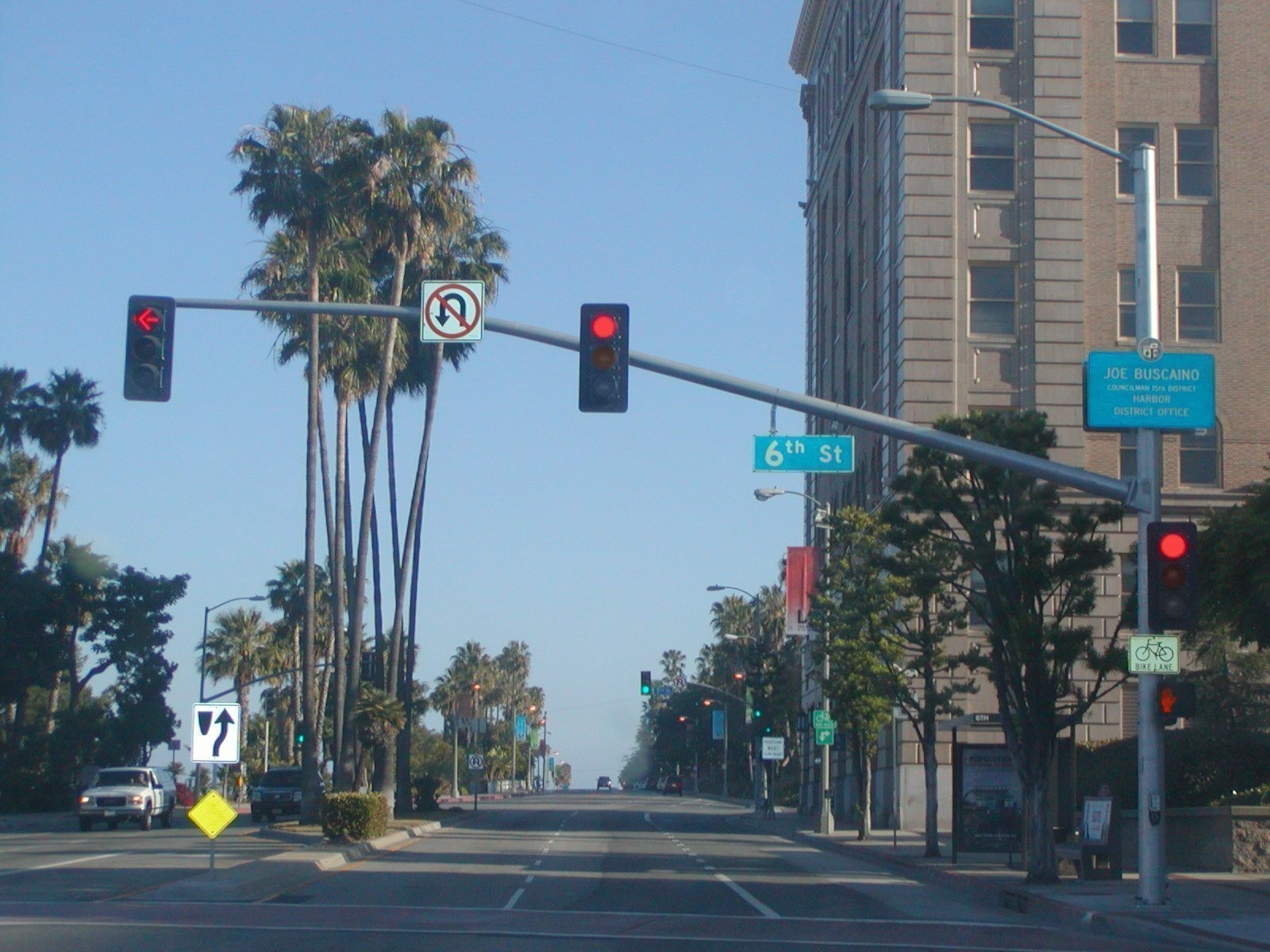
<box><xmin>134</xmin><ymin>773</ymin><xmax>148</xmax><ymax>785</ymax></box>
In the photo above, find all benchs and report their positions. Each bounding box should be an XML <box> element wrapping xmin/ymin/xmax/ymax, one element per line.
<box><xmin>1054</xmin><ymin>795</ymin><xmax>1123</xmax><ymax>880</ymax></box>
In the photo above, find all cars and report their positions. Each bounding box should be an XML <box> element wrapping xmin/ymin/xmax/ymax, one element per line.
<box><xmin>249</xmin><ymin>765</ymin><xmax>322</xmax><ymax>825</ymax></box>
<box><xmin>597</xmin><ymin>776</ymin><xmax>612</xmax><ymax>790</ymax></box>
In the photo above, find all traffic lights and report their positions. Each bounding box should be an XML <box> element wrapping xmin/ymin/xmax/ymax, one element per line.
<box><xmin>293</xmin><ymin>720</ymin><xmax>305</xmax><ymax>744</ymax></box>
<box><xmin>1155</xmin><ymin>678</ymin><xmax>1197</xmax><ymax>727</ymax></box>
<box><xmin>1144</xmin><ymin>521</ymin><xmax>1199</xmax><ymax>634</ymax></box>
<box><xmin>640</xmin><ymin>671</ymin><xmax>652</xmax><ymax>696</ymax></box>
<box><xmin>124</xmin><ymin>295</ymin><xmax>175</xmax><ymax>402</ymax></box>
<box><xmin>577</xmin><ymin>303</ymin><xmax>630</xmax><ymax>415</ymax></box>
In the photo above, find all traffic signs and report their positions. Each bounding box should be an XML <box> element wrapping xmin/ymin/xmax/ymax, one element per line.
<box><xmin>754</xmin><ymin>434</ymin><xmax>855</xmax><ymax>472</ymax></box>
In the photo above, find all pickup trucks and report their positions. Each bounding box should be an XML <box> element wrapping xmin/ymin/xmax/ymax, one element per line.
<box><xmin>78</xmin><ymin>767</ymin><xmax>175</xmax><ymax>831</ymax></box>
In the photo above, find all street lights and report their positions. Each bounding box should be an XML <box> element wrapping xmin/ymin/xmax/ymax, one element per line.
<box><xmin>467</xmin><ymin>684</ymin><xmax>565</xmax><ymax>812</ymax></box>
<box><xmin>199</xmin><ymin>595</ymin><xmax>340</xmax><ymax>702</ymax></box>
<box><xmin>676</xmin><ymin>486</ymin><xmax>879</xmax><ymax>838</ymax></box>
<box><xmin>868</xmin><ymin>81</ymin><xmax>1164</xmax><ymax>917</ymax></box>
